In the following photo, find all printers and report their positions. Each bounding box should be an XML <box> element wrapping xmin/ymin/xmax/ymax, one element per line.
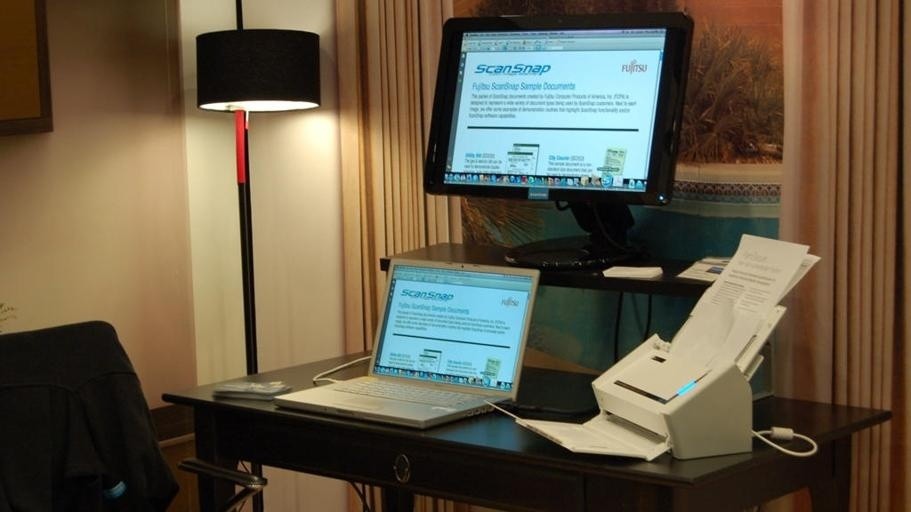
<box><xmin>484</xmin><ymin>305</ymin><xmax>788</xmax><ymax>463</ymax></box>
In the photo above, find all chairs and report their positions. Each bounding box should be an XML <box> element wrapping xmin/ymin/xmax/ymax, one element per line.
<box><xmin>0</xmin><ymin>321</ymin><xmax>267</xmax><ymax>512</ymax></box>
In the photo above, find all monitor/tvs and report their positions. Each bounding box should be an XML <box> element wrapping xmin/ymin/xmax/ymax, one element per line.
<box><xmin>423</xmin><ymin>11</ymin><xmax>695</xmax><ymax>272</ymax></box>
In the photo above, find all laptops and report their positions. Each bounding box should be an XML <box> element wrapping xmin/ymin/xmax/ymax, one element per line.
<box><xmin>271</xmin><ymin>256</ymin><xmax>542</xmax><ymax>433</ymax></box>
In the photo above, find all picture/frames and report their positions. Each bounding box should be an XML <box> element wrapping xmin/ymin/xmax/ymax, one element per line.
<box><xmin>0</xmin><ymin>0</ymin><xmax>53</xmax><ymax>137</ymax></box>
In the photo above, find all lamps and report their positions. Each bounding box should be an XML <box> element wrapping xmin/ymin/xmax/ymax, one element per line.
<box><xmin>197</xmin><ymin>1</ymin><xmax>322</xmax><ymax>512</ymax></box>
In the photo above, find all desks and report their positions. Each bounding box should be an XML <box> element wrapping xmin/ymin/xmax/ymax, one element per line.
<box><xmin>163</xmin><ymin>242</ymin><xmax>892</xmax><ymax>512</ymax></box>
